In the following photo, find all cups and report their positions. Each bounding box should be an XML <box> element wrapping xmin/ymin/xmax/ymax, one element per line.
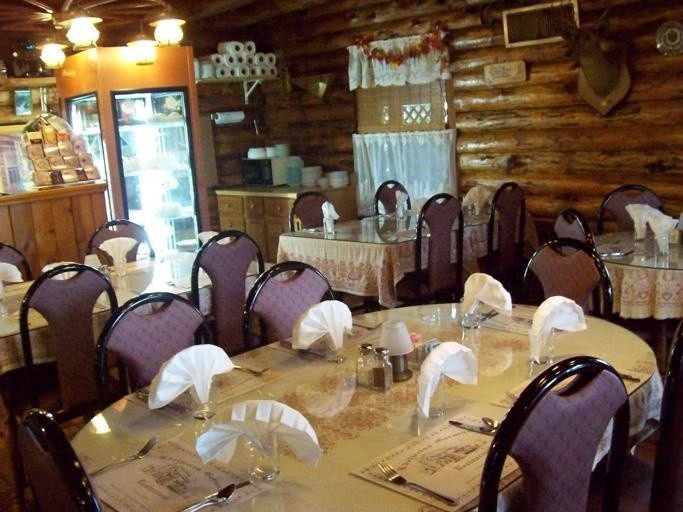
<box><xmin>633</xmin><ymin>223</ymin><xmax>647</xmax><ymax>243</ymax></box>
<box><xmin>529</xmin><ymin>328</ymin><xmax>554</xmax><ymax>365</ymax></box>
<box><xmin>245</xmin><ymin>427</ymin><xmax>281</xmax><ymax>481</ymax></box>
<box><xmin>422</xmin><ymin>370</ymin><xmax>446</xmax><ymax>417</ymax></box>
<box><xmin>322</xmin><ymin>329</ymin><xmax>344</xmax><ymax>362</ymax></box>
<box><xmin>323</xmin><ymin>200</ymin><xmax>484</xmax><ymax>237</ymax></box>
<box><xmin>653</xmin><ymin>233</ymin><xmax>670</xmax><ymax>258</ymax></box>
<box><xmin>113</xmin><ymin>258</ymin><xmax>127</xmax><ymax>278</ymax></box>
<box><xmin>461</xmin><ymin>298</ymin><xmax>479</xmax><ymax>329</ymax></box>
<box><xmin>190</xmin><ymin>377</ymin><xmax>217</xmax><ymax>419</ymax></box>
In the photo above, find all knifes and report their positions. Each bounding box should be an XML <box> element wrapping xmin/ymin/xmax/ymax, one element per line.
<box><xmin>180</xmin><ymin>480</ymin><xmax>250</xmax><ymax>512</ymax></box>
<box><xmin>448</xmin><ymin>416</ymin><xmax>497</xmax><ymax>435</ymax></box>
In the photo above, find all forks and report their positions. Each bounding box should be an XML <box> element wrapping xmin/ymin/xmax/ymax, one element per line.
<box><xmin>599</xmin><ymin>250</ymin><xmax>634</xmax><ymax>257</ymax></box>
<box><xmin>90</xmin><ymin>436</ymin><xmax>159</xmax><ymax>478</ymax></box>
<box><xmin>229</xmin><ymin>363</ymin><xmax>270</xmax><ymax>378</ymax></box>
<box><xmin>374</xmin><ymin>457</ymin><xmax>459</xmax><ymax>507</ymax></box>
<box><xmin>279</xmin><ymin>340</ymin><xmax>324</xmax><ymax>359</ymax></box>
<box><xmin>352</xmin><ymin>322</ymin><xmax>384</xmax><ymax>330</ymax></box>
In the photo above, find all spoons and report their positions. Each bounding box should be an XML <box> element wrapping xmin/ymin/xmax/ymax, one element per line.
<box><xmin>184</xmin><ymin>483</ymin><xmax>236</xmax><ymax>512</ymax></box>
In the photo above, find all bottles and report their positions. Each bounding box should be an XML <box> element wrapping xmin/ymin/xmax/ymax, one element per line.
<box><xmin>358</xmin><ymin>320</ymin><xmax>440</xmax><ymax>391</ymax></box>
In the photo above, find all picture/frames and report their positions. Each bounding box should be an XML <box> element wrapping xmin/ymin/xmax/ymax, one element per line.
<box><xmin>502</xmin><ymin>0</ymin><xmax>581</xmax><ymax>48</ymax></box>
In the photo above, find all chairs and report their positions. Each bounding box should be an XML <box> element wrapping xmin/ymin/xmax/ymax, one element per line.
<box><xmin>15</xmin><ymin>409</ymin><xmax>101</xmax><ymax>512</ymax></box>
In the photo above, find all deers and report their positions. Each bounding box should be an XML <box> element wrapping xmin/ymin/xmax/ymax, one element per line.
<box><xmin>554</xmin><ymin>7</ymin><xmax>632</xmax><ymax>116</ymax></box>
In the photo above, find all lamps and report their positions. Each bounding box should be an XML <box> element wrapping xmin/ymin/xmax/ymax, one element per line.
<box><xmin>126</xmin><ymin>20</ymin><xmax>158</xmax><ymax>65</ymax></box>
<box><xmin>58</xmin><ymin>0</ymin><xmax>104</xmax><ymax>52</ymax></box>
<box><xmin>149</xmin><ymin>3</ymin><xmax>186</xmax><ymax>48</ymax></box>
<box><xmin>35</xmin><ymin>27</ymin><xmax>69</xmax><ymax>70</ymax></box>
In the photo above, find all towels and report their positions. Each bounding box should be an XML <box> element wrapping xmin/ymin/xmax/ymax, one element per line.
<box><xmin>478</xmin><ymin>346</ymin><xmax>513</xmax><ymax>376</ymax></box>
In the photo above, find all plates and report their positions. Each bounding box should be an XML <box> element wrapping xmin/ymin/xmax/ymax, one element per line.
<box><xmin>247</xmin><ymin>143</ymin><xmax>350</xmax><ymax>189</ymax></box>
